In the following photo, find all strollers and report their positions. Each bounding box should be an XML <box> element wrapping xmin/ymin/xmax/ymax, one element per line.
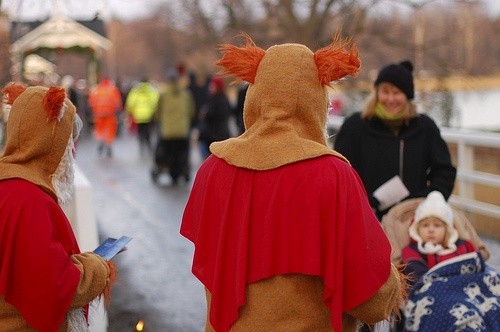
<box><xmin>374</xmin><ymin>197</ymin><xmax>489</xmax><ymax>332</ymax></box>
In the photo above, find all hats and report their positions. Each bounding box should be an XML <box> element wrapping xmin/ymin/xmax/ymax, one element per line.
<box><xmin>374</xmin><ymin>60</ymin><xmax>415</xmax><ymax>101</ymax></box>
<box><xmin>209</xmin><ymin>37</ymin><xmax>361</xmax><ymax>170</ymax></box>
<box><xmin>408</xmin><ymin>190</ymin><xmax>458</xmax><ymax>255</ymax></box>
<box><xmin>0</xmin><ymin>79</ymin><xmax>80</xmax><ymax>204</ymax></box>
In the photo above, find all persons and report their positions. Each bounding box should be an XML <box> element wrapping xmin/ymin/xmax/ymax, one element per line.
<box><xmin>400</xmin><ymin>192</ymin><xmax>476</xmax><ymax>285</ymax></box>
<box><xmin>24</xmin><ymin>54</ymin><xmax>250</xmax><ymax>188</ymax></box>
<box><xmin>178</xmin><ymin>33</ymin><xmax>413</xmax><ymax>332</ymax></box>
<box><xmin>333</xmin><ymin>60</ymin><xmax>458</xmax><ymax>332</ymax></box>
<box><xmin>0</xmin><ymin>81</ymin><xmax>119</xmax><ymax>332</ymax></box>
